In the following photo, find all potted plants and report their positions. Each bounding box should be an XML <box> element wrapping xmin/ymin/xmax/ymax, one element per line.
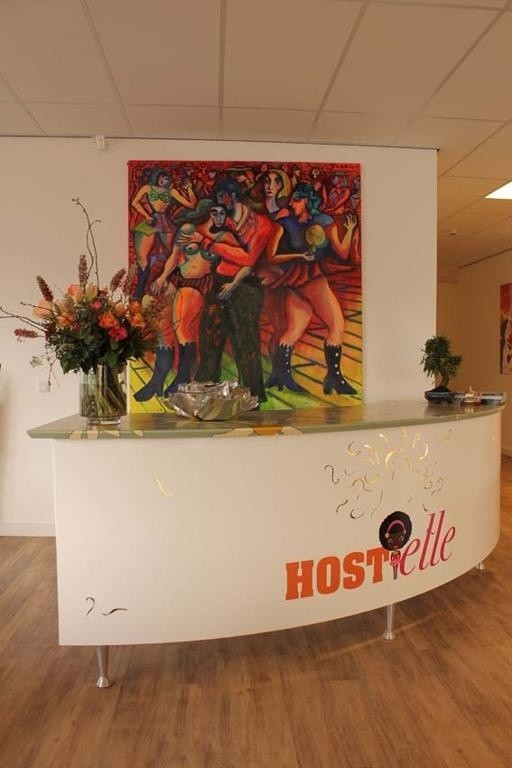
<box><xmin>417</xmin><ymin>334</ymin><xmax>464</xmax><ymax>403</ymax></box>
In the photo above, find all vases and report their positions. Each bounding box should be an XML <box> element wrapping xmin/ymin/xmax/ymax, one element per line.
<box><xmin>74</xmin><ymin>355</ymin><xmax>131</xmax><ymax>428</ymax></box>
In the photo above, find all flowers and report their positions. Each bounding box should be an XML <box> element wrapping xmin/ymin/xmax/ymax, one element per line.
<box><xmin>1</xmin><ymin>197</ymin><xmax>164</xmax><ymax>394</ymax></box>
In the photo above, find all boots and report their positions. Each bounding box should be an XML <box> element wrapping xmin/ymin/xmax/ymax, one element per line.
<box><xmin>321</xmin><ymin>342</ymin><xmax>358</xmax><ymax>395</ymax></box>
<box><xmin>276</xmin><ymin>341</ymin><xmax>308</xmax><ymax>394</ymax></box>
<box><xmin>236</xmin><ymin>359</ymin><xmax>268</xmax><ymax>404</ymax></box>
<box><xmin>133</xmin><ymin>345</ymin><xmax>174</xmax><ymax>402</ymax></box>
<box><xmin>164</xmin><ymin>342</ymin><xmax>198</xmax><ymax>397</ymax></box>
